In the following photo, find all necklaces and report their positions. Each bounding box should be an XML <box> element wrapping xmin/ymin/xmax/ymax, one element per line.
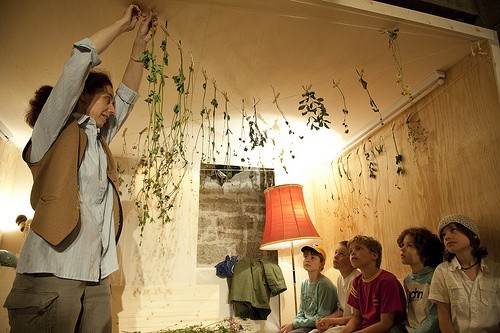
<box><xmin>461</xmin><ymin>261</ymin><xmax>480</xmax><ymax>272</ymax></box>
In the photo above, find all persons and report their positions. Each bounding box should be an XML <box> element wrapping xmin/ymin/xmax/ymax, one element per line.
<box><xmin>428</xmin><ymin>214</ymin><xmax>500</xmax><ymax>333</ymax></box>
<box><xmin>338</xmin><ymin>235</ymin><xmax>407</xmax><ymax>333</ymax></box>
<box><xmin>308</xmin><ymin>241</ymin><xmax>360</xmax><ymax>333</ymax></box>
<box><xmin>382</xmin><ymin>227</ymin><xmax>444</xmax><ymax>333</ymax></box>
<box><xmin>2</xmin><ymin>4</ymin><xmax>159</xmax><ymax>333</ymax></box>
<box><xmin>276</xmin><ymin>245</ymin><xmax>338</xmax><ymax>333</ymax></box>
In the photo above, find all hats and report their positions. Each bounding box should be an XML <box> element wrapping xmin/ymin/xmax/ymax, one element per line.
<box><xmin>437</xmin><ymin>214</ymin><xmax>480</xmax><ymax>243</ymax></box>
<box><xmin>301</xmin><ymin>243</ymin><xmax>326</xmax><ymax>261</ymax></box>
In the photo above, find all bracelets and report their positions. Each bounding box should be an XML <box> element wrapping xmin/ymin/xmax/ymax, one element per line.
<box><xmin>129</xmin><ymin>56</ymin><xmax>143</xmax><ymax>62</ymax></box>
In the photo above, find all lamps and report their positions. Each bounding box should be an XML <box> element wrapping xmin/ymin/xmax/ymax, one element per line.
<box><xmin>259</xmin><ymin>182</ymin><xmax>323</xmax><ymax>321</ymax></box>
<box><xmin>330</xmin><ymin>71</ymin><xmax>446</xmax><ymax>161</ymax></box>
<box><xmin>0</xmin><ymin>120</ymin><xmax>13</xmax><ymax>142</ymax></box>
